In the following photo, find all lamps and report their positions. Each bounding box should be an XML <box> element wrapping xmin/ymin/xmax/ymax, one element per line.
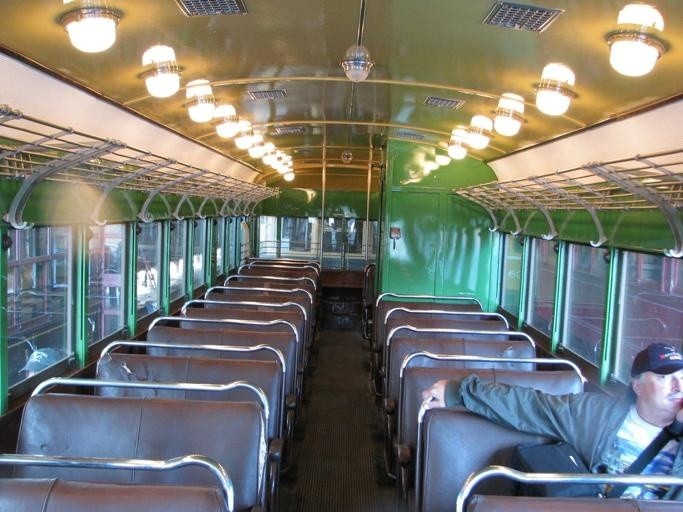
<box><xmin>603</xmin><ymin>3</ymin><xmax>671</xmax><ymax>79</ymax></box>
<box><xmin>341</xmin><ymin>150</ymin><xmax>353</xmax><ymax>164</ymax></box>
<box><xmin>136</xmin><ymin>44</ymin><xmax>184</xmax><ymax>100</ymax></box>
<box><xmin>53</xmin><ymin>0</ymin><xmax>125</xmax><ymax>54</ymax></box>
<box><xmin>404</xmin><ymin>115</ymin><xmax>493</xmax><ymax>183</ymax></box>
<box><xmin>493</xmin><ymin>93</ymin><xmax>528</xmax><ymax>137</ymax></box>
<box><xmin>531</xmin><ymin>62</ymin><xmax>579</xmax><ymax>118</ymax></box>
<box><xmin>340</xmin><ymin>0</ymin><xmax>377</xmax><ymax>82</ymax></box>
<box><xmin>181</xmin><ymin>78</ymin><xmax>220</xmax><ymax>123</ymax></box>
<box><xmin>214</xmin><ymin>104</ymin><xmax>296</xmax><ymax>183</ymax></box>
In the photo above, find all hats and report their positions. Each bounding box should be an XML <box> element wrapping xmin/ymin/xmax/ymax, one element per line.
<box><xmin>631</xmin><ymin>342</ymin><xmax>682</xmax><ymax>376</ymax></box>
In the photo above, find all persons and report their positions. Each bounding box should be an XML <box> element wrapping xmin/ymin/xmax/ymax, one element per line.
<box><xmin>348</xmin><ymin>219</ymin><xmax>357</xmax><ymax>249</ymax></box>
<box><xmin>421</xmin><ymin>342</ymin><xmax>683</xmax><ymax>503</ymax></box>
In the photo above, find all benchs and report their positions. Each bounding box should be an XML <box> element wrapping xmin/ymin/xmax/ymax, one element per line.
<box><xmin>414</xmin><ymin>394</ymin><xmax>602</xmax><ymax>512</ymax></box>
<box><xmin>394</xmin><ymin>349</ymin><xmax>586</xmax><ymax>501</ymax></box>
<box><xmin>371</xmin><ymin>292</ymin><xmax>485</xmax><ymax>387</ymax></box>
<box><xmin>456</xmin><ymin>463</ymin><xmax>683</xmax><ymax>512</ymax></box>
<box><xmin>181</xmin><ymin>258</ymin><xmax>320</xmax><ymax>395</ymax></box>
<box><xmin>374</xmin><ymin>307</ymin><xmax>510</xmax><ymax>407</ymax></box>
<box><xmin>93</xmin><ymin>340</ymin><xmax>285</xmax><ymax>512</ymax></box>
<box><xmin>14</xmin><ymin>377</ymin><xmax>269</xmax><ymax>512</ymax></box>
<box><xmin>0</xmin><ymin>451</ymin><xmax>237</xmax><ymax>511</ymax></box>
<box><xmin>144</xmin><ymin>316</ymin><xmax>299</xmax><ymax>471</ymax></box>
<box><xmin>384</xmin><ymin>325</ymin><xmax>537</xmax><ymax>444</ymax></box>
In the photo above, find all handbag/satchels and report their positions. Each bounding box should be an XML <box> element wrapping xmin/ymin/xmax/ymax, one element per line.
<box><xmin>510</xmin><ymin>441</ymin><xmax>600</xmax><ymax>497</ymax></box>
